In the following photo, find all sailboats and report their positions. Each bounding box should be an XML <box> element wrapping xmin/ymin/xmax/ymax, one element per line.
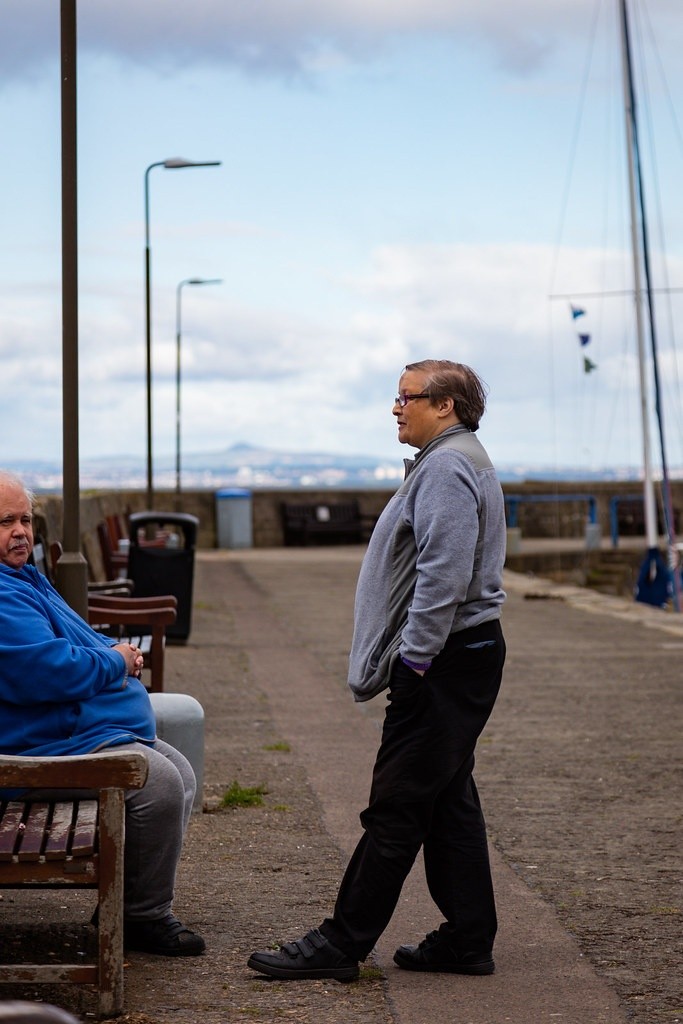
<box><xmin>549</xmin><ymin>1</ymin><xmax>683</xmax><ymax>613</ymax></box>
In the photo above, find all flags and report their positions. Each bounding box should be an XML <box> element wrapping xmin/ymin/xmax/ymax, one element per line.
<box><xmin>585</xmin><ymin>359</ymin><xmax>596</xmax><ymax>372</ymax></box>
<box><xmin>579</xmin><ymin>334</ymin><xmax>589</xmax><ymax>345</ymax></box>
<box><xmin>572</xmin><ymin>306</ymin><xmax>586</xmax><ymax>317</ymax></box>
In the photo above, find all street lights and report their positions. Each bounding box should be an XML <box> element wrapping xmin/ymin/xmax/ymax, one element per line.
<box><xmin>144</xmin><ymin>154</ymin><xmax>223</xmax><ymax>508</ymax></box>
<box><xmin>176</xmin><ymin>277</ymin><xmax>219</xmax><ymax>506</ymax></box>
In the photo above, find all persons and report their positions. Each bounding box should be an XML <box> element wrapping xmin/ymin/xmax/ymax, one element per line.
<box><xmin>249</xmin><ymin>360</ymin><xmax>508</xmax><ymax>977</ymax></box>
<box><xmin>1</xmin><ymin>469</ymin><xmax>206</xmax><ymax>955</ymax></box>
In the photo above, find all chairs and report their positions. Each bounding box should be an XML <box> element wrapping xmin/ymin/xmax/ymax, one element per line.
<box><xmin>0</xmin><ymin>503</ymin><xmax>179</xmax><ymax>1016</ymax></box>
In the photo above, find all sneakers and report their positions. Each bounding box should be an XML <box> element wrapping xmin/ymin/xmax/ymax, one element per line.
<box><xmin>247</xmin><ymin>928</ymin><xmax>360</xmax><ymax>982</ymax></box>
<box><xmin>91</xmin><ymin>902</ymin><xmax>99</xmax><ymax>925</ymax></box>
<box><xmin>124</xmin><ymin>911</ymin><xmax>206</xmax><ymax>956</ymax></box>
<box><xmin>393</xmin><ymin>930</ymin><xmax>495</xmax><ymax>975</ymax></box>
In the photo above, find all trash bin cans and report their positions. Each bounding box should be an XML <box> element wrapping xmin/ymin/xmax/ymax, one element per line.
<box><xmin>124</xmin><ymin>512</ymin><xmax>200</xmax><ymax>644</ymax></box>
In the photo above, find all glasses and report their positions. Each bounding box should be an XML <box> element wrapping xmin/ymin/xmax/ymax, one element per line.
<box><xmin>394</xmin><ymin>394</ymin><xmax>432</xmax><ymax>407</ymax></box>
